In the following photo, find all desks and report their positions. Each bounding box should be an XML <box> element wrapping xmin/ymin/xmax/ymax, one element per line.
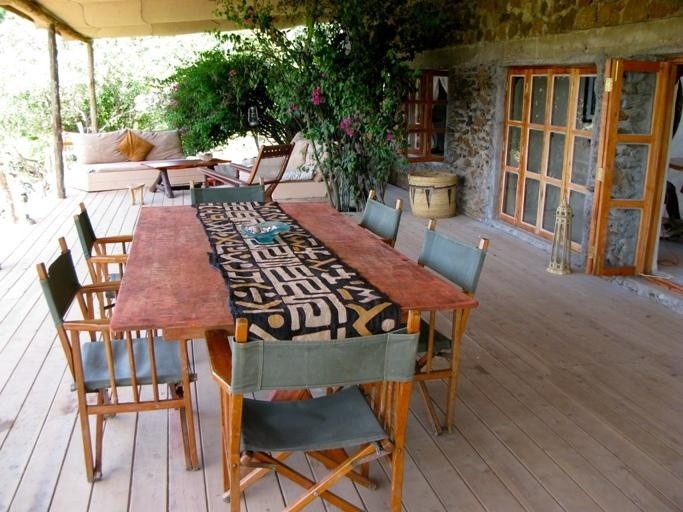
<box><xmin>141</xmin><ymin>158</ymin><xmax>232</xmax><ymax>198</ymax></box>
<box><xmin>109</xmin><ymin>201</ymin><xmax>479</xmax><ymax>436</ymax></box>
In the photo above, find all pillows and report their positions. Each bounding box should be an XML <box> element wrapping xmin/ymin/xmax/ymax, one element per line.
<box><xmin>113</xmin><ymin>130</ymin><xmax>155</xmax><ymax>162</ymax></box>
<box><xmin>71</xmin><ymin>128</ymin><xmax>129</xmax><ymax>164</ymax></box>
<box><xmin>125</xmin><ymin>127</ymin><xmax>186</xmax><ymax>160</ymax></box>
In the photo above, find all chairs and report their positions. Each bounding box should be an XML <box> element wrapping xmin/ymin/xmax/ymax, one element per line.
<box><xmin>36</xmin><ymin>236</ymin><xmax>200</xmax><ymax>483</ymax></box>
<box><xmin>411</xmin><ymin>218</ymin><xmax>489</xmax><ymax>435</ymax></box>
<box><xmin>199</xmin><ymin>142</ymin><xmax>295</xmax><ymax>201</ymax></box>
<box><xmin>205</xmin><ymin>309</ymin><xmax>421</xmax><ymax>512</ymax></box>
<box><xmin>188</xmin><ymin>177</ymin><xmax>266</xmax><ymax>207</ymax></box>
<box><xmin>73</xmin><ymin>201</ymin><xmax>133</xmax><ymax>318</ymax></box>
<box><xmin>357</xmin><ymin>190</ymin><xmax>403</xmax><ymax>249</ymax></box>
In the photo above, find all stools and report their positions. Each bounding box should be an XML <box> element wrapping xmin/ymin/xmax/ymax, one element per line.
<box><xmin>129</xmin><ymin>183</ymin><xmax>145</xmax><ymax>205</ymax></box>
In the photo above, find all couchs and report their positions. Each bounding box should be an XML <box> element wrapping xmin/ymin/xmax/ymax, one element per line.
<box><xmin>62</xmin><ymin>129</ymin><xmax>213</xmax><ymax>192</ymax></box>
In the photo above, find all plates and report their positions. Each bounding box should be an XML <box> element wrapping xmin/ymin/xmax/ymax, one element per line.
<box><xmin>240</xmin><ymin>220</ymin><xmax>290</xmax><ymax>244</ymax></box>
